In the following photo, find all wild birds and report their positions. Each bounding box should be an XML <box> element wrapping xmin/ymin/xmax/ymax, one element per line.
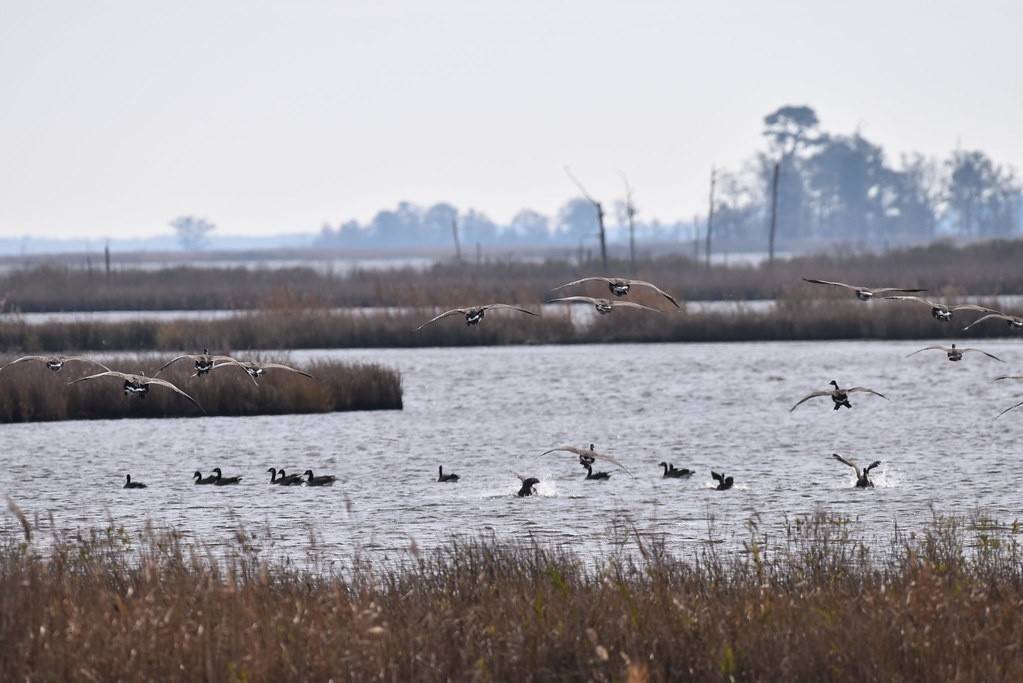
<box><xmin>582</xmin><ymin>463</ymin><xmax>617</xmax><ymax>482</ymax></box>
<box><xmin>994</xmin><ymin>400</ymin><xmax>1023</xmax><ymax>421</ymax></box>
<box><xmin>905</xmin><ymin>343</ymin><xmax>1007</xmax><ymax>364</ymax></box>
<box><xmin>544</xmin><ymin>295</ymin><xmax>662</xmax><ymax>314</ymax></box>
<box><xmin>962</xmin><ymin>313</ymin><xmax>1023</xmax><ymax>332</ymax></box>
<box><xmin>789</xmin><ymin>379</ymin><xmax>891</xmax><ymax>413</ymax></box>
<box><xmin>832</xmin><ymin>452</ymin><xmax>882</xmax><ymax>489</ymax></box>
<box><xmin>122</xmin><ymin>473</ymin><xmax>148</xmax><ymax>489</ymax></box>
<box><xmin>802</xmin><ymin>278</ymin><xmax>930</xmax><ymax>302</ymax></box>
<box><xmin>66</xmin><ymin>370</ymin><xmax>209</xmax><ymax>415</ymax></box>
<box><xmin>266</xmin><ymin>467</ymin><xmax>307</xmax><ymax>487</ymax></box>
<box><xmin>416</xmin><ymin>302</ymin><xmax>541</xmax><ymax>332</ymax></box>
<box><xmin>0</xmin><ymin>352</ymin><xmax>112</xmax><ymax>377</ymax></box>
<box><xmin>192</xmin><ymin>470</ymin><xmax>218</xmax><ymax>485</ymax></box>
<box><xmin>210</xmin><ymin>467</ymin><xmax>244</xmax><ymax>486</ymax></box>
<box><xmin>704</xmin><ymin>469</ymin><xmax>736</xmax><ymax>492</ymax></box>
<box><xmin>551</xmin><ymin>275</ymin><xmax>681</xmax><ymax>309</ymax></box>
<box><xmin>506</xmin><ymin>466</ymin><xmax>540</xmax><ymax>499</ymax></box>
<box><xmin>190</xmin><ymin>360</ymin><xmax>316</xmax><ymax>381</ymax></box>
<box><xmin>879</xmin><ymin>294</ymin><xmax>1008</xmax><ymax>323</ymax></box>
<box><xmin>992</xmin><ymin>376</ymin><xmax>1023</xmax><ymax>383</ymax></box>
<box><xmin>536</xmin><ymin>443</ymin><xmax>634</xmax><ymax>480</ymax></box>
<box><xmin>437</xmin><ymin>464</ymin><xmax>462</xmax><ymax>484</ymax></box>
<box><xmin>302</xmin><ymin>469</ymin><xmax>338</xmax><ymax>487</ymax></box>
<box><xmin>152</xmin><ymin>347</ymin><xmax>260</xmax><ymax>388</ymax></box>
<box><xmin>657</xmin><ymin>461</ymin><xmax>696</xmax><ymax>479</ymax></box>
